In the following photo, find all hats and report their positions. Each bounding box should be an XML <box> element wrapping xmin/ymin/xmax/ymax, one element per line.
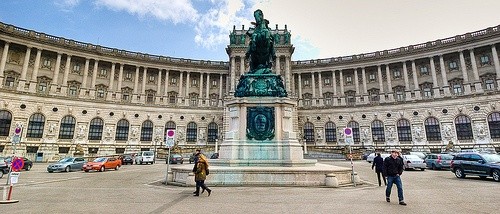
<box><xmin>391</xmin><ymin>149</ymin><xmax>399</xmax><ymax>153</ymax></box>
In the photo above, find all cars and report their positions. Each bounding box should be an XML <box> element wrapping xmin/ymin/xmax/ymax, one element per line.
<box><xmin>401</xmin><ymin>155</ymin><xmax>426</xmax><ymax>172</ymax></box>
<box><xmin>120</xmin><ymin>156</ymin><xmax>133</xmax><ymax>165</ymax></box>
<box><xmin>367</xmin><ymin>153</ymin><xmax>378</xmax><ymax>164</ymax></box>
<box><xmin>165</xmin><ymin>152</ymin><xmax>183</xmax><ymax>164</ymax></box>
<box><xmin>404</xmin><ymin>152</ymin><xmax>426</xmax><ymax>159</ymax></box>
<box><xmin>0</xmin><ymin>156</ymin><xmax>8</xmax><ymax>179</ymax></box>
<box><xmin>362</xmin><ymin>153</ymin><xmax>370</xmax><ymax>160</ymax></box>
<box><xmin>189</xmin><ymin>153</ymin><xmax>197</xmax><ymax>164</ymax></box>
<box><xmin>47</xmin><ymin>157</ymin><xmax>87</xmax><ymax>173</ymax></box>
<box><xmin>5</xmin><ymin>157</ymin><xmax>33</xmax><ymax>172</ymax></box>
<box><xmin>82</xmin><ymin>157</ymin><xmax>122</xmax><ymax>172</ymax></box>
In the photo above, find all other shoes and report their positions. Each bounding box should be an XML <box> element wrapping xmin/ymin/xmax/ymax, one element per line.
<box><xmin>207</xmin><ymin>189</ymin><xmax>211</xmax><ymax>196</ymax></box>
<box><xmin>193</xmin><ymin>191</ymin><xmax>197</xmax><ymax>193</ymax></box>
<box><xmin>193</xmin><ymin>193</ymin><xmax>199</xmax><ymax>196</ymax></box>
<box><xmin>202</xmin><ymin>189</ymin><xmax>205</xmax><ymax>193</ymax></box>
<box><xmin>399</xmin><ymin>202</ymin><xmax>406</xmax><ymax>205</ymax></box>
<box><xmin>386</xmin><ymin>198</ymin><xmax>390</xmax><ymax>202</ymax></box>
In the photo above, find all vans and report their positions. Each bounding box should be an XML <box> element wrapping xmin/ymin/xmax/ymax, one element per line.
<box><xmin>135</xmin><ymin>151</ymin><xmax>154</xmax><ymax>165</ymax></box>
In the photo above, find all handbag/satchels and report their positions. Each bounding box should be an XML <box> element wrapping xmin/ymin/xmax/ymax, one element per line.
<box><xmin>206</xmin><ymin>169</ymin><xmax>209</xmax><ymax>175</ymax></box>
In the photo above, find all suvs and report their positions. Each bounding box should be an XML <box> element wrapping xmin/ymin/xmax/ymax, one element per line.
<box><xmin>423</xmin><ymin>153</ymin><xmax>454</xmax><ymax>170</ymax></box>
<box><xmin>451</xmin><ymin>152</ymin><xmax>500</xmax><ymax>182</ymax></box>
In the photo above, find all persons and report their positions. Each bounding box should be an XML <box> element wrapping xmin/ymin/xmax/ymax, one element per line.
<box><xmin>372</xmin><ymin>151</ymin><xmax>404</xmax><ymax>186</ymax></box>
<box><xmin>192</xmin><ymin>150</ymin><xmax>212</xmax><ymax>197</ymax></box>
<box><xmin>383</xmin><ymin>150</ymin><xmax>407</xmax><ymax>205</ymax></box>
